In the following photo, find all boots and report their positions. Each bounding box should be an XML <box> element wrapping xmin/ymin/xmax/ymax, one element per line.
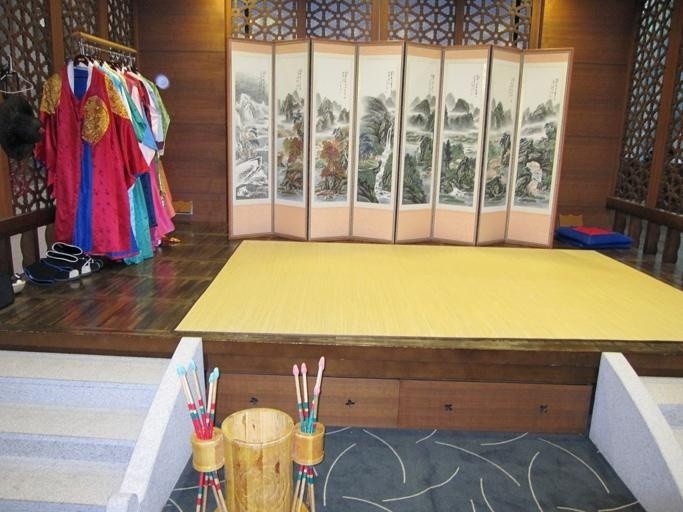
<box><xmin>23</xmin><ymin>241</ymin><xmax>105</xmax><ymax>286</ymax></box>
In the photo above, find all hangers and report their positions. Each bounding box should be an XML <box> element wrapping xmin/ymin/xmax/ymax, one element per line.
<box><xmin>74</xmin><ymin>40</ymin><xmax>138</xmax><ymax>68</ymax></box>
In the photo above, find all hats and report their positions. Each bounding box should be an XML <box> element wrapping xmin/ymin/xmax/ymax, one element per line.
<box><xmin>0</xmin><ymin>95</ymin><xmax>44</xmax><ymax>161</ymax></box>
